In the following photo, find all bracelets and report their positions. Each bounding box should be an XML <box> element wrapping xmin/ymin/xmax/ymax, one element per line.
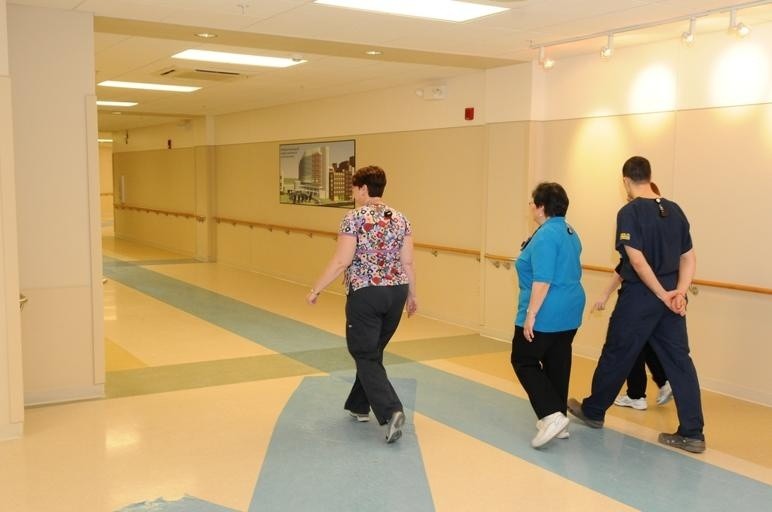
<box><xmin>526</xmin><ymin>310</ymin><xmax>538</xmax><ymax>317</ymax></box>
<box><xmin>310</xmin><ymin>288</ymin><xmax>319</xmax><ymax>296</ymax></box>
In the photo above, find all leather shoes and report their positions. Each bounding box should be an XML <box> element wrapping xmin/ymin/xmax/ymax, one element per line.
<box><xmin>566</xmin><ymin>398</ymin><xmax>604</xmax><ymax>429</ymax></box>
<box><xmin>658</xmin><ymin>432</ymin><xmax>706</xmax><ymax>453</ymax></box>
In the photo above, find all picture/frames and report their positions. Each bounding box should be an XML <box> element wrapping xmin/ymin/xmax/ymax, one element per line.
<box><xmin>279</xmin><ymin>139</ymin><xmax>356</xmax><ymax>209</ymax></box>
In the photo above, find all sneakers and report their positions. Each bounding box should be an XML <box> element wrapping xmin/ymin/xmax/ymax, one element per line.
<box><xmin>536</xmin><ymin>414</ymin><xmax>570</xmax><ymax>439</ymax></box>
<box><xmin>655</xmin><ymin>380</ymin><xmax>673</xmax><ymax>406</ymax></box>
<box><xmin>531</xmin><ymin>412</ymin><xmax>569</xmax><ymax>448</ymax></box>
<box><xmin>385</xmin><ymin>411</ymin><xmax>405</xmax><ymax>442</ymax></box>
<box><xmin>349</xmin><ymin>411</ymin><xmax>370</xmax><ymax>422</ymax></box>
<box><xmin>613</xmin><ymin>395</ymin><xmax>648</xmax><ymax>410</ymax></box>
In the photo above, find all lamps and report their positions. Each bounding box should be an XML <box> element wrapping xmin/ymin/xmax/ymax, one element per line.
<box><xmin>537</xmin><ymin>11</ymin><xmax>752</xmax><ymax>70</ymax></box>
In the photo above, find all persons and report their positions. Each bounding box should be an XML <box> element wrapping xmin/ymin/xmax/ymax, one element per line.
<box><xmin>307</xmin><ymin>164</ymin><xmax>419</xmax><ymax>446</ymax></box>
<box><xmin>564</xmin><ymin>154</ymin><xmax>707</xmax><ymax>456</ymax></box>
<box><xmin>510</xmin><ymin>178</ymin><xmax>585</xmax><ymax>452</ymax></box>
<box><xmin>590</xmin><ymin>182</ymin><xmax>673</xmax><ymax>413</ymax></box>
